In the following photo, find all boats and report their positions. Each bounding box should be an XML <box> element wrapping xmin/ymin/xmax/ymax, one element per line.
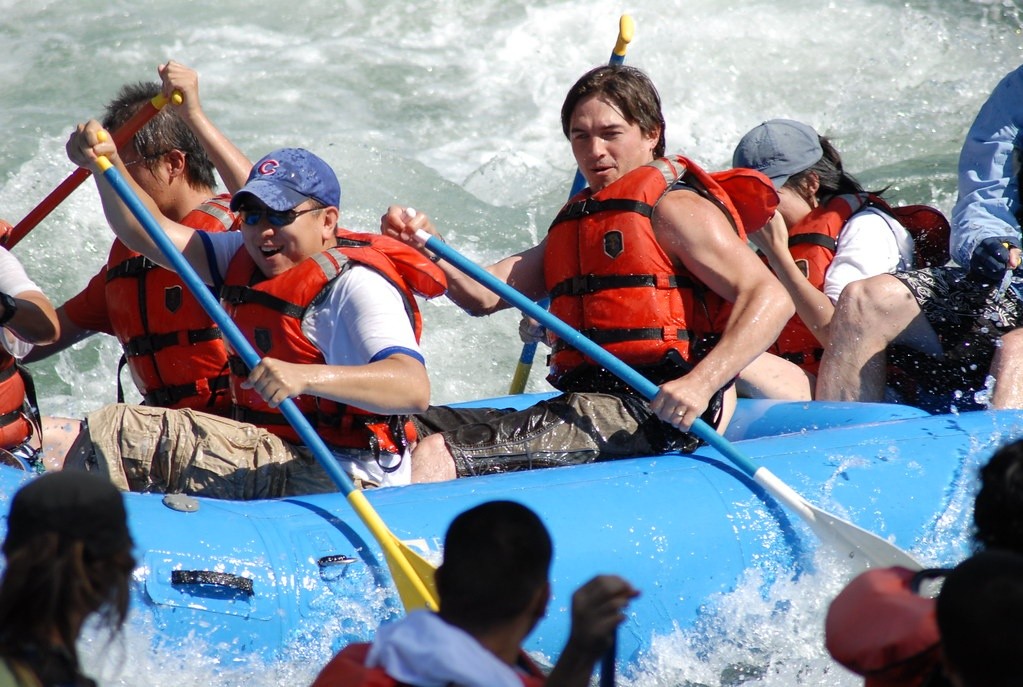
<box><xmin>1</xmin><ymin>392</ymin><xmax>1023</xmax><ymax>687</ymax></box>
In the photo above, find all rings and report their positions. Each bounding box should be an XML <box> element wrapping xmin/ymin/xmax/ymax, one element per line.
<box><xmin>673</xmin><ymin>410</ymin><xmax>685</xmax><ymax>418</ymax></box>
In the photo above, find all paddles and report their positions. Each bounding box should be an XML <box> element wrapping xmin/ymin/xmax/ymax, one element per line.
<box><xmin>402</xmin><ymin>205</ymin><xmax>941</xmax><ymax>598</ymax></box>
<box><xmin>598</xmin><ymin>622</ymin><xmax>624</xmax><ymax>686</ymax></box>
<box><xmin>2</xmin><ymin>85</ymin><xmax>183</xmax><ymax>252</ymax></box>
<box><xmin>508</xmin><ymin>14</ymin><xmax>635</xmax><ymax>397</ymax></box>
<box><xmin>83</xmin><ymin>129</ymin><xmax>441</xmax><ymax>615</ymax></box>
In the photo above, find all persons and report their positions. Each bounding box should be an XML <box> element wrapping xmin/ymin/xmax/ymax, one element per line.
<box><xmin>0</xmin><ymin>245</ymin><xmax>61</xmax><ymax>475</ymax></box>
<box><xmin>731</xmin><ymin>117</ymin><xmax>917</xmax><ymax>402</ymax></box>
<box><xmin>62</xmin><ymin>118</ymin><xmax>430</xmax><ymax>503</ymax></box>
<box><xmin>0</xmin><ymin>464</ymin><xmax>137</xmax><ymax>687</ymax></box>
<box><xmin>20</xmin><ymin>60</ymin><xmax>254</xmax><ymax>476</ymax></box>
<box><xmin>310</xmin><ymin>500</ymin><xmax>641</xmax><ymax>687</ymax></box>
<box><xmin>866</xmin><ymin>437</ymin><xmax>1023</xmax><ymax>687</ymax></box>
<box><xmin>815</xmin><ymin>63</ymin><xmax>1023</xmax><ymax>412</ymax></box>
<box><xmin>380</xmin><ymin>64</ymin><xmax>796</xmax><ymax>484</ymax></box>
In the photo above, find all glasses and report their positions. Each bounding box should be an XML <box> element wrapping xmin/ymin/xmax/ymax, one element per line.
<box><xmin>237</xmin><ymin>201</ymin><xmax>327</xmax><ymax>227</ymax></box>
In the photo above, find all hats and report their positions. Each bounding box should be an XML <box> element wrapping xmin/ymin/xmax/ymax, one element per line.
<box><xmin>732</xmin><ymin>118</ymin><xmax>824</xmax><ymax>190</ymax></box>
<box><xmin>229</xmin><ymin>147</ymin><xmax>341</xmax><ymax>212</ymax></box>
